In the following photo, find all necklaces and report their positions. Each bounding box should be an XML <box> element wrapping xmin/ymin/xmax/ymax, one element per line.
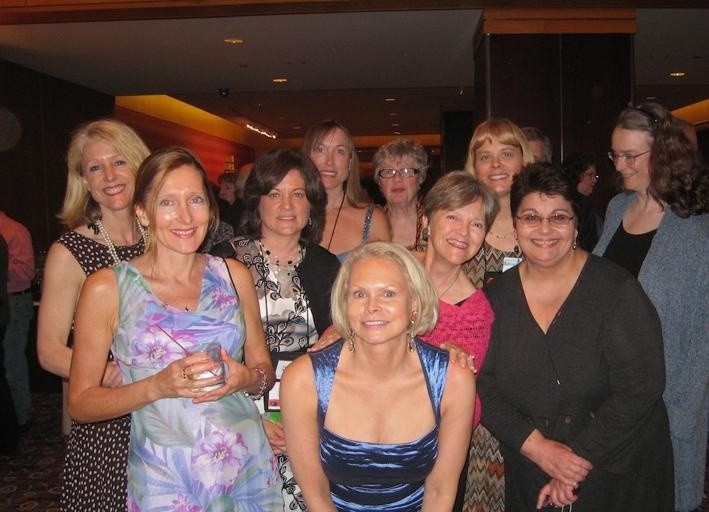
<box><xmin>147</xmin><ymin>257</ymin><xmax>205</xmax><ymax>311</ymax></box>
<box><xmin>96</xmin><ymin>215</ymin><xmax>148</xmax><ymax>264</ymax></box>
<box><xmin>420</xmin><ymin>253</ymin><xmax>461</xmax><ymax>299</ymax></box>
<box><xmin>488</xmin><ymin>228</ymin><xmax>516</xmax><ymax>240</ymax></box>
<box><xmin>252</xmin><ymin>236</ymin><xmax>308</xmax><ymax>277</ymax></box>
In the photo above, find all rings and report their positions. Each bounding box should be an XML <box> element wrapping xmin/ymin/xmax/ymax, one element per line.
<box><xmin>183</xmin><ymin>368</ymin><xmax>187</xmax><ymax>380</ymax></box>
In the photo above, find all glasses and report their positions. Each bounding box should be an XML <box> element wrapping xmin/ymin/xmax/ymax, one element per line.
<box><xmin>606</xmin><ymin>150</ymin><xmax>651</xmax><ymax>164</ymax></box>
<box><xmin>514</xmin><ymin>212</ymin><xmax>576</xmax><ymax>229</ymax></box>
<box><xmin>585</xmin><ymin>171</ymin><xmax>603</xmax><ymax>182</ymax></box>
<box><xmin>378</xmin><ymin>167</ymin><xmax>420</xmax><ymax>179</ymax></box>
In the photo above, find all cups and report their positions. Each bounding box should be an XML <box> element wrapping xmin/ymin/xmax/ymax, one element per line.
<box><xmin>181</xmin><ymin>342</ymin><xmax>226</xmax><ymax>392</ymax></box>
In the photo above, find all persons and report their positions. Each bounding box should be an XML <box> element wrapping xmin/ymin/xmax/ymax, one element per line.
<box><xmin>39</xmin><ymin>121</ymin><xmax>153</xmax><ymax>512</ymax></box>
<box><xmin>676</xmin><ymin>117</ymin><xmax>698</xmax><ymax>149</ymax></box>
<box><xmin>476</xmin><ymin>164</ymin><xmax>675</xmax><ymax>512</ymax></box>
<box><xmin>210</xmin><ymin>145</ymin><xmax>341</xmax><ymax>456</ymax></box>
<box><xmin>218</xmin><ymin>172</ymin><xmax>235</xmax><ymax>204</ymax></box>
<box><xmin>199</xmin><ymin>217</ymin><xmax>232</xmax><ymax>254</ymax></box>
<box><xmin>411</xmin><ymin>170</ymin><xmax>494</xmax><ymax>429</ymax></box>
<box><xmin>591</xmin><ymin>102</ymin><xmax>709</xmax><ymax>512</ymax></box>
<box><xmin>563</xmin><ymin>154</ymin><xmax>604</xmax><ymax>238</ymax></box>
<box><xmin>302</xmin><ymin>118</ymin><xmax>390</xmax><ymax>264</ymax></box>
<box><xmin>0</xmin><ymin>235</ymin><xmax>20</xmax><ymax>459</ymax></box>
<box><xmin>374</xmin><ymin>138</ymin><xmax>429</xmax><ymax>250</ymax></box>
<box><xmin>1</xmin><ymin>210</ymin><xmax>34</xmax><ymax>433</ymax></box>
<box><xmin>234</xmin><ymin>162</ymin><xmax>256</xmax><ymax>196</ymax></box>
<box><xmin>66</xmin><ymin>145</ymin><xmax>283</xmax><ymax>512</ymax></box>
<box><xmin>279</xmin><ymin>242</ymin><xmax>475</xmax><ymax>512</ymax></box>
<box><xmin>463</xmin><ymin>116</ymin><xmax>535</xmax><ymax>289</ymax></box>
<box><xmin>522</xmin><ymin>126</ymin><xmax>598</xmax><ymax>251</ymax></box>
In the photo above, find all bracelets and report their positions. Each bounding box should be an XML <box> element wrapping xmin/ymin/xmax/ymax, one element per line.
<box><xmin>243</xmin><ymin>367</ymin><xmax>268</xmax><ymax>401</ymax></box>
